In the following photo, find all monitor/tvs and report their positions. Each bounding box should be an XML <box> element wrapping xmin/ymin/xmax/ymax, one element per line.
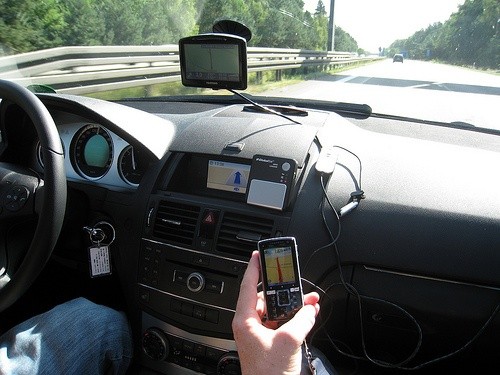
<box><xmin>179</xmin><ymin>33</ymin><xmax>248</xmax><ymax>90</ymax></box>
<box><xmin>206</xmin><ymin>160</ymin><xmax>251</xmax><ymax>194</ymax></box>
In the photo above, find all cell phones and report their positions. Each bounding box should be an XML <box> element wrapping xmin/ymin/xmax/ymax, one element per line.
<box><xmin>257</xmin><ymin>236</ymin><xmax>304</xmax><ymax>322</ymax></box>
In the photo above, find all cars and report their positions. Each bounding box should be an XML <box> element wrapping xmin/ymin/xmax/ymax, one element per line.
<box><xmin>392</xmin><ymin>53</ymin><xmax>404</xmax><ymax>63</ymax></box>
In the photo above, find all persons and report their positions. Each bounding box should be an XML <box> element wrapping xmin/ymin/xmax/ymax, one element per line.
<box><xmin>0</xmin><ymin>295</ymin><xmax>135</xmax><ymax>375</ymax></box>
<box><xmin>232</xmin><ymin>250</ymin><xmax>338</xmax><ymax>375</ymax></box>
